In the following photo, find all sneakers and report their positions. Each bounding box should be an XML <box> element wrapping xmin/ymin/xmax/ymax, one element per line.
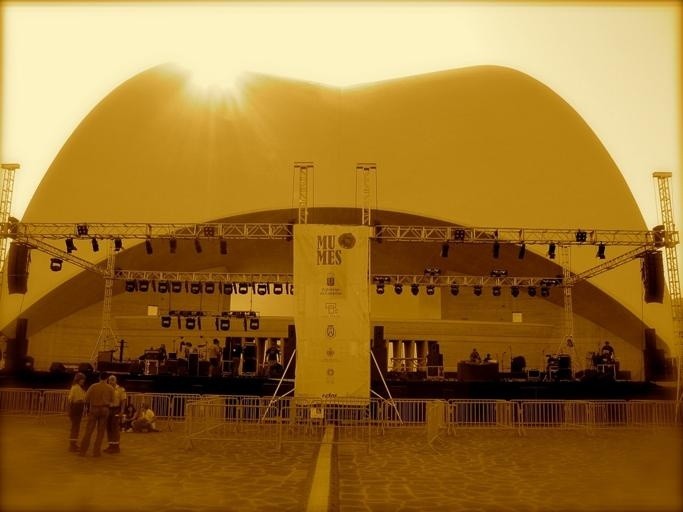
<box><xmin>141</xmin><ymin>428</ymin><xmax>148</xmax><ymax>432</ymax></box>
<box><xmin>127</xmin><ymin>429</ymin><xmax>133</xmax><ymax>433</ymax></box>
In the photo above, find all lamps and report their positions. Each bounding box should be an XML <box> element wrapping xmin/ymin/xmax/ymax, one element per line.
<box><xmin>369</xmin><ymin>267</ymin><xmax>566</xmax><ymax>298</ymax></box>
<box><xmin>50</xmin><ymin>258</ymin><xmax>63</xmax><ymax>272</ymax></box>
<box><xmin>16</xmin><ymin>223</ymin><xmax>294</xmax><ymax>256</ymax></box>
<box><xmin>369</xmin><ymin>222</ymin><xmax>680</xmax><ymax>263</ymax></box>
<box><xmin>125</xmin><ymin>272</ymin><xmax>294</xmax><ymax>296</ymax></box>
<box><xmin>160</xmin><ymin>310</ymin><xmax>260</xmax><ymax>333</ymax></box>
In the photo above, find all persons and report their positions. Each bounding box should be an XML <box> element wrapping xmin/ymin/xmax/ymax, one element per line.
<box><xmin>103</xmin><ymin>374</ymin><xmax>127</xmax><ymax>453</ymax></box>
<box><xmin>119</xmin><ymin>404</ymin><xmax>137</xmax><ymax>432</ymax></box>
<box><xmin>484</xmin><ymin>354</ymin><xmax>492</xmax><ymax>363</ymax></box>
<box><xmin>175</xmin><ymin>339</ymin><xmax>280</xmax><ymax>381</ymax></box>
<box><xmin>69</xmin><ymin>373</ymin><xmax>87</xmax><ymax>451</ymax></box>
<box><xmin>470</xmin><ymin>348</ymin><xmax>481</xmax><ymax>363</ymax></box>
<box><xmin>601</xmin><ymin>341</ymin><xmax>616</xmax><ymax>358</ymax></box>
<box><xmin>131</xmin><ymin>403</ymin><xmax>156</xmax><ymax>432</ymax></box>
<box><xmin>79</xmin><ymin>372</ymin><xmax>115</xmax><ymax>457</ymax></box>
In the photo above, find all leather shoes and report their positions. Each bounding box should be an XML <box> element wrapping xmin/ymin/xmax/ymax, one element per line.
<box><xmin>69</xmin><ymin>447</ymin><xmax>80</xmax><ymax>452</ymax></box>
<box><xmin>104</xmin><ymin>446</ymin><xmax>112</xmax><ymax>451</ymax></box>
<box><xmin>108</xmin><ymin>448</ymin><xmax>120</xmax><ymax>453</ymax></box>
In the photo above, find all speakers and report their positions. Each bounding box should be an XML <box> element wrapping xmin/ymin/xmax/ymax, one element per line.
<box><xmin>641</xmin><ymin>254</ymin><xmax>664</xmax><ymax>304</ymax></box>
<box><xmin>8</xmin><ymin>245</ymin><xmax>30</xmax><ymax>294</ymax></box>
